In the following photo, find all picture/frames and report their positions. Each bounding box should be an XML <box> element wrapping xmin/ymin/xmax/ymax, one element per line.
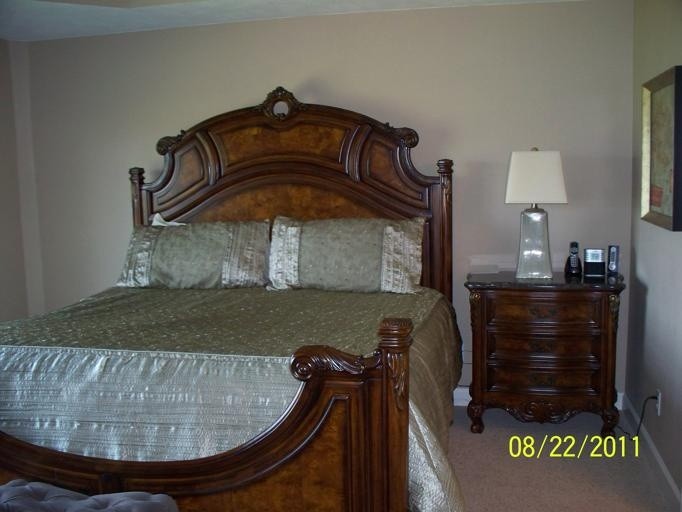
<box><xmin>641</xmin><ymin>64</ymin><xmax>682</xmax><ymax>232</ymax></box>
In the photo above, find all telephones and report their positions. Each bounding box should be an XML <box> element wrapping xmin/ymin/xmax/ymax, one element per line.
<box><xmin>564</xmin><ymin>242</ymin><xmax>582</xmax><ymax>279</ymax></box>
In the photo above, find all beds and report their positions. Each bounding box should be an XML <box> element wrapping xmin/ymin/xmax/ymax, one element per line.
<box><xmin>0</xmin><ymin>84</ymin><xmax>464</xmax><ymax>512</ymax></box>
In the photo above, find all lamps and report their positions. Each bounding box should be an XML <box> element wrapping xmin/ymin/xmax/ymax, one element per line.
<box><xmin>504</xmin><ymin>147</ymin><xmax>568</xmax><ymax>280</ymax></box>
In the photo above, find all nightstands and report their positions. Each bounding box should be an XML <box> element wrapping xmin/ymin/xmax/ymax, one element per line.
<box><xmin>465</xmin><ymin>273</ymin><xmax>626</xmax><ymax>440</ymax></box>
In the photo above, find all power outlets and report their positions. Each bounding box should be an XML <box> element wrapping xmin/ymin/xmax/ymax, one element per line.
<box><xmin>654</xmin><ymin>389</ymin><xmax>662</xmax><ymax>416</ymax></box>
